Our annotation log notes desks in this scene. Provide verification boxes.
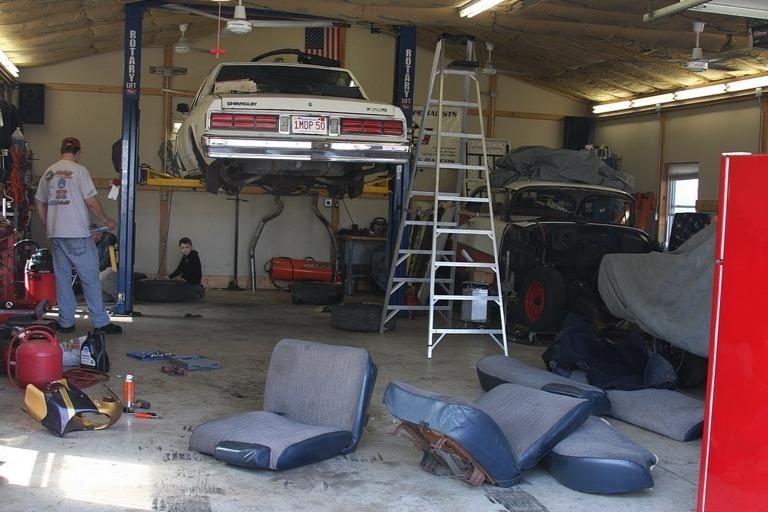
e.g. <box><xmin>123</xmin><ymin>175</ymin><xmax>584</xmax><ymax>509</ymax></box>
<box><xmin>336</xmin><ymin>235</ymin><xmax>390</xmax><ymax>297</ymax></box>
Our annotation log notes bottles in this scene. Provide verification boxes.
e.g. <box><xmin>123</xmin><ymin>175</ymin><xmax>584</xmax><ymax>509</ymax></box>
<box><xmin>89</xmin><ymin>227</ymin><xmax>112</xmax><ymax>236</ymax></box>
<box><xmin>405</xmin><ymin>283</ymin><xmax>418</xmax><ymax>319</ymax></box>
<box><xmin>123</xmin><ymin>373</ymin><xmax>135</xmax><ymax>413</ymax></box>
<box><xmin>336</xmin><ymin>270</ymin><xmax>342</xmax><ymax>285</ymax></box>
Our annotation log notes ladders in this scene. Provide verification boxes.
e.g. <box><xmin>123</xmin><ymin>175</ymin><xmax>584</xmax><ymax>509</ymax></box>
<box><xmin>378</xmin><ymin>33</ymin><xmax>508</xmax><ymax>358</ymax></box>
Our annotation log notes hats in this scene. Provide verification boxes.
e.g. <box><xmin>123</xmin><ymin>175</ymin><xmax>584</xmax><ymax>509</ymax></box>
<box><xmin>62</xmin><ymin>137</ymin><xmax>82</xmax><ymax>147</ymax></box>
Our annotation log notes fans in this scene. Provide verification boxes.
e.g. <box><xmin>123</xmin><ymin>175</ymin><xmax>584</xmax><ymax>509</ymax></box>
<box><xmin>142</xmin><ymin>24</ymin><xmax>209</xmax><ymax>53</ymax></box>
<box><xmin>641</xmin><ymin>21</ymin><xmax>757</xmax><ymax>72</ymax></box>
<box><xmin>163</xmin><ymin>0</ymin><xmax>333</xmax><ymax>34</ymax></box>
<box><xmin>461</xmin><ymin>43</ymin><xmax>530</xmax><ymax>74</ymax></box>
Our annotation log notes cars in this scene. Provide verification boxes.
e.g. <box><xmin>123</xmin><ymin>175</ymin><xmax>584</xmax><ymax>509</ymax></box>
<box><xmin>450</xmin><ymin>182</ymin><xmax>656</xmax><ymax>333</ymax></box>
<box><xmin>169</xmin><ymin>61</ymin><xmax>413</xmax><ymax>200</ymax></box>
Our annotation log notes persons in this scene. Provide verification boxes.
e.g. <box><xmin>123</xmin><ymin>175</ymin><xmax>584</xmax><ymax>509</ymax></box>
<box><xmin>88</xmin><ymin>223</ymin><xmax>120</xmax><ymax>300</ymax></box>
<box><xmin>166</xmin><ymin>237</ymin><xmax>205</xmax><ymax>298</ymax></box>
<box><xmin>34</xmin><ymin>137</ymin><xmax>122</xmax><ymax>335</ymax></box>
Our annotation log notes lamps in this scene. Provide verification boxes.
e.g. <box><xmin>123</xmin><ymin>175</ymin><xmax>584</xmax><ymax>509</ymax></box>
<box><xmin>0</xmin><ymin>54</ymin><xmax>19</xmax><ymax>81</ymax></box>
<box><xmin>590</xmin><ymin>75</ymin><xmax>768</xmax><ymax>117</ymax></box>
<box><xmin>642</xmin><ymin>0</ymin><xmax>714</xmax><ymax>21</ymax></box>
<box><xmin>460</xmin><ymin>0</ymin><xmax>504</xmax><ymax>20</ymax></box>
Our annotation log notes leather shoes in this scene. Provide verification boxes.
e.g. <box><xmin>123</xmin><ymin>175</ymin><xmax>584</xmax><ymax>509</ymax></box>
<box><xmin>61</xmin><ymin>324</ymin><xmax>76</xmax><ymax>333</ymax></box>
<box><xmin>95</xmin><ymin>322</ymin><xmax>121</xmax><ymax>334</ymax></box>
